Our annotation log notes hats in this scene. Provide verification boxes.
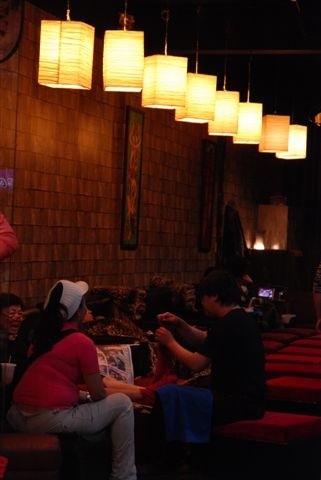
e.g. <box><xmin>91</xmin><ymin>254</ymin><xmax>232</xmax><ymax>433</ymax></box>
<box><xmin>45</xmin><ymin>279</ymin><xmax>89</xmax><ymax>320</ymax></box>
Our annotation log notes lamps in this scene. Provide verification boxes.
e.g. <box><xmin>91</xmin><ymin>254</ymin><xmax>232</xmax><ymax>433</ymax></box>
<box><xmin>207</xmin><ymin>27</ymin><xmax>240</xmax><ymax>136</ymax></box>
<box><xmin>175</xmin><ymin>2</ymin><xmax>217</xmax><ymax>124</ymax></box>
<box><xmin>38</xmin><ymin>0</ymin><xmax>95</xmax><ymax>90</ymax></box>
<box><xmin>102</xmin><ymin>0</ymin><xmax>145</xmax><ymax>92</ymax></box>
<box><xmin>274</xmin><ymin>114</ymin><xmax>308</xmax><ymax>160</ymax></box>
<box><xmin>141</xmin><ymin>10</ymin><xmax>188</xmax><ymax>110</ymax></box>
<box><xmin>232</xmin><ymin>54</ymin><xmax>263</xmax><ymax>145</ymax></box>
<box><xmin>259</xmin><ymin>101</ymin><xmax>292</xmax><ymax>153</ymax></box>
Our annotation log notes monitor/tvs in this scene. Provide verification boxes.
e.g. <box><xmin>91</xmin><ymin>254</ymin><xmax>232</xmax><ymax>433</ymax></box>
<box><xmin>258</xmin><ymin>287</ymin><xmax>275</xmax><ymax>301</ymax></box>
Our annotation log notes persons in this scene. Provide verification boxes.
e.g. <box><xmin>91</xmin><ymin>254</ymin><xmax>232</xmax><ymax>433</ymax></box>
<box><xmin>154</xmin><ymin>270</ymin><xmax>268</xmax><ymax>473</ymax></box>
<box><xmin>0</xmin><ymin>292</ymin><xmax>24</xmax><ymax>425</ymax></box>
<box><xmin>81</xmin><ymin>310</ymin><xmax>190</xmax><ymax>405</ymax></box>
<box><xmin>8</xmin><ymin>280</ymin><xmax>137</xmax><ymax>480</ymax></box>
<box><xmin>312</xmin><ymin>262</ymin><xmax>321</xmax><ymax>330</ymax></box>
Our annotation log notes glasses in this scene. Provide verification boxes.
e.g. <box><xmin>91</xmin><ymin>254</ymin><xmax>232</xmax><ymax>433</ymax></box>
<box><xmin>0</xmin><ymin>312</ymin><xmax>25</xmax><ymax>321</ymax></box>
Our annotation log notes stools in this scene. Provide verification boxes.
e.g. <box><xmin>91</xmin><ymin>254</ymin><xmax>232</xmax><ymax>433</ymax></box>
<box><xmin>0</xmin><ymin>327</ymin><xmax>321</xmax><ymax>480</ymax></box>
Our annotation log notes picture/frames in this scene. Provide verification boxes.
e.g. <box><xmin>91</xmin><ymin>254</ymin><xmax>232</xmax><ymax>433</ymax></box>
<box><xmin>198</xmin><ymin>138</ymin><xmax>217</xmax><ymax>252</ymax></box>
<box><xmin>120</xmin><ymin>105</ymin><xmax>145</xmax><ymax>249</ymax></box>
